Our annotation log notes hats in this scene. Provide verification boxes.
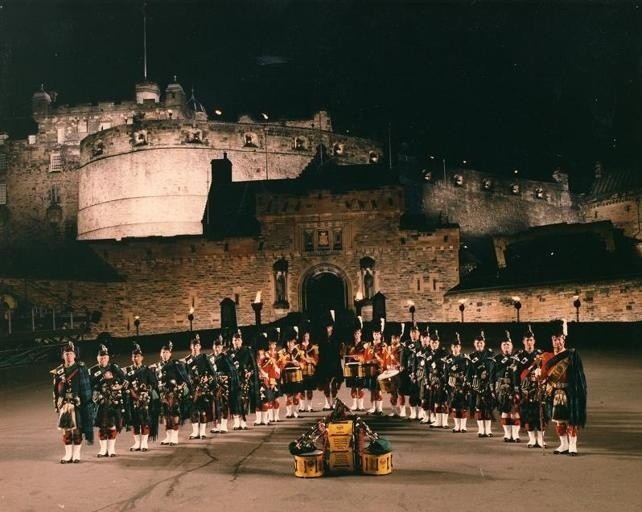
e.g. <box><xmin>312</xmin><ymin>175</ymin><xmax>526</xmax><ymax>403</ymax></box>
<box><xmin>551</xmin><ymin>326</ymin><xmax>563</xmax><ymax>336</ymax></box>
<box><xmin>132</xmin><ymin>348</ymin><xmax>142</xmax><ymax>355</ymax></box>
<box><xmin>355</xmin><ymin>326</ymin><xmax>401</xmax><ymax>336</ymax></box>
<box><xmin>523</xmin><ymin>331</ymin><xmax>534</xmax><ymax>338</ymax></box>
<box><xmin>476</xmin><ymin>335</ymin><xmax>483</xmax><ymax>341</ymax></box>
<box><xmin>502</xmin><ymin>338</ymin><xmax>511</xmax><ymax>342</ymax></box>
<box><xmin>162</xmin><ymin>345</ymin><xmax>170</xmax><ymax>350</ymax></box>
<box><xmin>257</xmin><ymin>320</ymin><xmax>332</xmax><ymax>349</ymax></box>
<box><xmin>410</xmin><ymin>326</ymin><xmax>439</xmax><ymax>341</ymax></box>
<box><xmin>213</xmin><ymin>340</ymin><xmax>222</xmax><ymax>345</ymax></box>
<box><xmin>190</xmin><ymin>339</ymin><xmax>200</xmax><ymax>344</ymax></box>
<box><xmin>233</xmin><ymin>333</ymin><xmax>241</xmax><ymax>338</ymax></box>
<box><xmin>97</xmin><ymin>349</ymin><xmax>108</xmax><ymax>356</ymax></box>
<box><xmin>454</xmin><ymin>339</ymin><xmax>461</xmax><ymax>345</ymax></box>
<box><xmin>62</xmin><ymin>343</ymin><xmax>74</xmax><ymax>352</ymax></box>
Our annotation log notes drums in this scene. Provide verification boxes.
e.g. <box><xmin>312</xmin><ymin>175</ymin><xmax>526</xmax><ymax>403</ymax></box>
<box><xmin>344</xmin><ymin>362</ymin><xmax>359</xmax><ymax>379</ymax></box>
<box><xmin>293</xmin><ymin>449</ymin><xmax>324</xmax><ymax>479</ymax></box>
<box><xmin>358</xmin><ymin>361</ymin><xmax>379</xmax><ymax>379</ymax></box>
<box><xmin>301</xmin><ymin>359</ymin><xmax>317</xmax><ymax>377</ymax></box>
<box><xmin>284</xmin><ymin>366</ymin><xmax>304</xmax><ymax>386</ymax></box>
<box><xmin>377</xmin><ymin>368</ymin><xmax>401</xmax><ymax>395</ymax></box>
<box><xmin>358</xmin><ymin>447</ymin><xmax>394</xmax><ymax>476</ymax></box>
<box><xmin>323</xmin><ymin>419</ymin><xmax>365</xmax><ymax>477</ymax></box>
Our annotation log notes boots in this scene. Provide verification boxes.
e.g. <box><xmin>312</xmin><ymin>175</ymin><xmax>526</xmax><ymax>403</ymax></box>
<box><xmin>527</xmin><ymin>430</ymin><xmax>536</xmax><ymax>448</ymax></box>
<box><xmin>527</xmin><ymin>430</ymin><xmax>547</xmax><ymax>448</ymax></box>
<box><xmin>350</xmin><ymin>396</ymin><xmax>492</xmax><ymax>443</ymax></box>
<box><xmin>254</xmin><ymin>408</ymin><xmax>281</xmax><ymax>425</ymax></box>
<box><xmin>568</xmin><ymin>433</ymin><xmax>577</xmax><ymax>456</ymax></box>
<box><xmin>72</xmin><ymin>444</ymin><xmax>82</xmax><ymax>463</ymax></box>
<box><xmin>107</xmin><ymin>438</ymin><xmax>117</xmax><ymax>457</ymax></box>
<box><xmin>129</xmin><ymin>433</ymin><xmax>140</xmax><ymax>451</ymax></box>
<box><xmin>285</xmin><ymin>399</ymin><xmax>313</xmax><ymax>418</ymax></box>
<box><xmin>189</xmin><ymin>422</ymin><xmax>208</xmax><ymax>439</ymax></box>
<box><xmin>233</xmin><ymin>413</ymin><xmax>248</xmax><ymax>430</ymax></box>
<box><xmin>512</xmin><ymin>425</ymin><xmax>520</xmax><ymax>443</ymax></box>
<box><xmin>537</xmin><ymin>430</ymin><xmax>547</xmax><ymax>448</ymax></box>
<box><xmin>323</xmin><ymin>395</ymin><xmax>336</xmax><ymax>410</ymax></box>
<box><xmin>554</xmin><ymin>435</ymin><xmax>568</xmax><ymax>454</ymax></box>
<box><xmin>97</xmin><ymin>439</ymin><xmax>107</xmax><ymax>457</ymax></box>
<box><xmin>141</xmin><ymin>433</ymin><xmax>149</xmax><ymax>452</ymax></box>
<box><xmin>502</xmin><ymin>424</ymin><xmax>511</xmax><ymax>441</ymax></box>
<box><xmin>210</xmin><ymin>417</ymin><xmax>228</xmax><ymax>433</ymax></box>
<box><xmin>160</xmin><ymin>429</ymin><xmax>178</xmax><ymax>445</ymax></box>
<box><xmin>61</xmin><ymin>444</ymin><xmax>72</xmax><ymax>464</ymax></box>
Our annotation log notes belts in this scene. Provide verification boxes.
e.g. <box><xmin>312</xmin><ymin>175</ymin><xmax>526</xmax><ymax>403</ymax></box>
<box><xmin>553</xmin><ymin>383</ymin><xmax>569</xmax><ymax>388</ymax></box>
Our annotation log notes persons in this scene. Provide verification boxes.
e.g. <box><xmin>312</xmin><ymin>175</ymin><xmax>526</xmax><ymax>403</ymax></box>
<box><xmin>148</xmin><ymin>333</ymin><xmax>258</xmax><ymax>446</ymax></box>
<box><xmin>468</xmin><ymin>331</ymin><xmax>586</xmax><ymax>456</ymax></box>
<box><xmin>49</xmin><ymin>345</ymin><xmax>92</xmax><ymax>463</ymax></box>
<box><xmin>87</xmin><ymin>343</ymin><xmax>160</xmax><ymax>457</ymax></box>
<box><xmin>316</xmin><ymin>324</ymin><xmax>345</xmax><ymax>411</ymax></box>
<box><xmin>254</xmin><ymin>331</ymin><xmax>319</xmax><ymax>425</ymax></box>
<box><xmin>345</xmin><ymin>329</ymin><xmax>406</xmax><ymax>418</ymax></box>
<box><xmin>397</xmin><ymin>325</ymin><xmax>471</xmax><ymax>432</ymax></box>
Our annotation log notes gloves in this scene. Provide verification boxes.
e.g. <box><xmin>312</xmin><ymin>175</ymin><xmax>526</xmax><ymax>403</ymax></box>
<box><xmin>534</xmin><ymin>367</ymin><xmax>541</xmax><ymax>378</ymax></box>
